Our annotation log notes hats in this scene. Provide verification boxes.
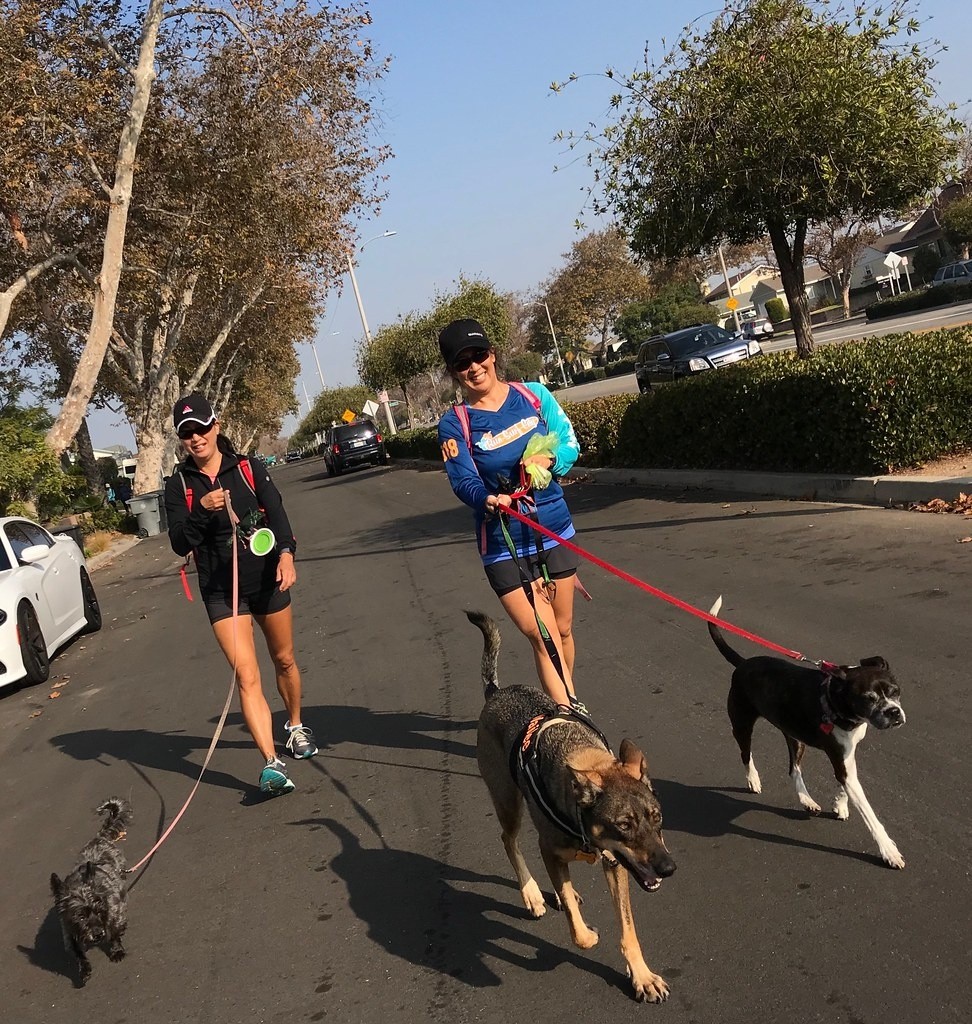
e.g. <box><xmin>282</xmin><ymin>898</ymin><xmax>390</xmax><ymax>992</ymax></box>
<box><xmin>438</xmin><ymin>318</ymin><xmax>490</xmax><ymax>368</ymax></box>
<box><xmin>173</xmin><ymin>395</ymin><xmax>216</xmax><ymax>434</ymax></box>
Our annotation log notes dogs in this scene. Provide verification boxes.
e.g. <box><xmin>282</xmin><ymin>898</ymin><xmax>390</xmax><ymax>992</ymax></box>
<box><xmin>461</xmin><ymin>608</ymin><xmax>677</xmax><ymax>1005</ymax></box>
<box><xmin>49</xmin><ymin>795</ymin><xmax>133</xmax><ymax>984</ymax></box>
<box><xmin>707</xmin><ymin>593</ymin><xmax>908</xmax><ymax>872</ymax></box>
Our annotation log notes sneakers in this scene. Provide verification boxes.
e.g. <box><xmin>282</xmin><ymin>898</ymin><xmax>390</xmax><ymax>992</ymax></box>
<box><xmin>284</xmin><ymin>720</ymin><xmax>319</xmax><ymax>760</ymax></box>
<box><xmin>258</xmin><ymin>758</ymin><xmax>296</xmax><ymax>797</ymax></box>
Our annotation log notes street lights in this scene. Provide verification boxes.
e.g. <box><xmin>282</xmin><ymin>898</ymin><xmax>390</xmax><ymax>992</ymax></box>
<box><xmin>523</xmin><ymin>301</ymin><xmax>568</xmax><ymax>388</ymax></box>
<box><xmin>300</xmin><ymin>228</ymin><xmax>398</xmax><ymax>434</ymax></box>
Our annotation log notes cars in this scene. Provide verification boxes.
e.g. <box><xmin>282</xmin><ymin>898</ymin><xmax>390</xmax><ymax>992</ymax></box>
<box><xmin>0</xmin><ymin>516</ymin><xmax>102</xmax><ymax>690</ymax></box>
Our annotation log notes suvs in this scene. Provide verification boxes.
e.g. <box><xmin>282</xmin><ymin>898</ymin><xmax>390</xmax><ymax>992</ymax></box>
<box><xmin>634</xmin><ymin>321</ymin><xmax>763</xmax><ymax>395</ymax></box>
<box><xmin>323</xmin><ymin>419</ymin><xmax>388</xmax><ymax>474</ymax></box>
<box><xmin>284</xmin><ymin>448</ymin><xmax>301</xmax><ymax>462</ymax></box>
<box><xmin>742</xmin><ymin>319</ymin><xmax>774</xmax><ymax>341</ymax></box>
<box><xmin>933</xmin><ymin>258</ymin><xmax>972</xmax><ymax>285</ymax></box>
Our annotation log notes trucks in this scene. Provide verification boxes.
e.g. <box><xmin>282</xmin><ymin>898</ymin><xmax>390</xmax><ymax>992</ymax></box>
<box><xmin>256</xmin><ymin>454</ymin><xmax>276</xmax><ymax>467</ymax></box>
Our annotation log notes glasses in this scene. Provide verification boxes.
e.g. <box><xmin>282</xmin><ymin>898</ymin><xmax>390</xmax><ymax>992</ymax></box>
<box><xmin>176</xmin><ymin>418</ymin><xmax>215</xmax><ymax>439</ymax></box>
<box><xmin>450</xmin><ymin>346</ymin><xmax>490</xmax><ymax>372</ymax></box>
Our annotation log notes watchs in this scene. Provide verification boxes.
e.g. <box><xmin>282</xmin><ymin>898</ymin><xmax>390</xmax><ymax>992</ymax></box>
<box><xmin>280</xmin><ymin>547</ymin><xmax>295</xmax><ymax>557</ymax></box>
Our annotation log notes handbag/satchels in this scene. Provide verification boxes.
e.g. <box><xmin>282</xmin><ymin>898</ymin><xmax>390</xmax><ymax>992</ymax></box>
<box><xmin>520</xmin><ymin>430</ymin><xmax>559</xmax><ymax>489</ymax></box>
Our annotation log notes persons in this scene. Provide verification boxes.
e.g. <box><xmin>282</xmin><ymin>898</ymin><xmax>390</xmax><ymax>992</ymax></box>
<box><xmin>117</xmin><ymin>480</ymin><xmax>131</xmax><ymax>516</ymax></box>
<box><xmin>164</xmin><ymin>396</ymin><xmax>318</xmax><ymax>795</ymax></box>
<box><xmin>439</xmin><ymin>319</ymin><xmax>580</xmax><ymax>714</ymax></box>
<box><xmin>104</xmin><ymin>483</ymin><xmax>117</xmax><ymax>514</ymax></box>
